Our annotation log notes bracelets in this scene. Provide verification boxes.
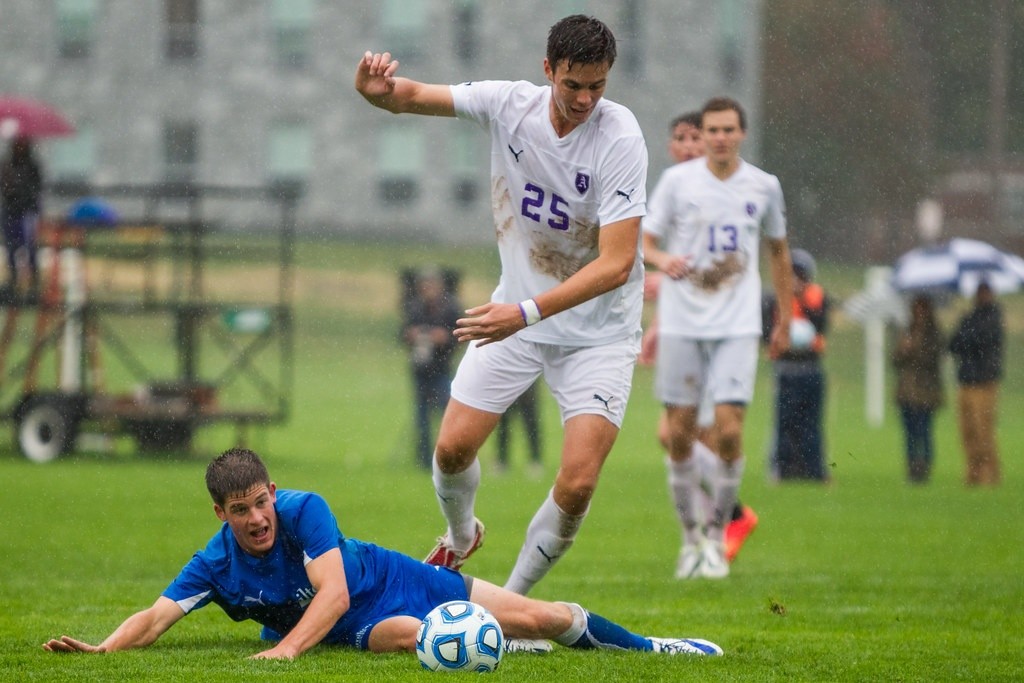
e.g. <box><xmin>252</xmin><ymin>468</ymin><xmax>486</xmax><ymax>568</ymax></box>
<box><xmin>518</xmin><ymin>298</ymin><xmax>542</xmax><ymax>327</ymax></box>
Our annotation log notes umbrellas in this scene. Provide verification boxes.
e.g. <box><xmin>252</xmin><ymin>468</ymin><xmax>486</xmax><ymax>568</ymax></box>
<box><xmin>0</xmin><ymin>94</ymin><xmax>79</xmax><ymax>138</ymax></box>
<box><xmin>886</xmin><ymin>236</ymin><xmax>1024</xmax><ymax>311</ymax></box>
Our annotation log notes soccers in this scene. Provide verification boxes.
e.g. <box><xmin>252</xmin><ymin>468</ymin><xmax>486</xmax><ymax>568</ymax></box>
<box><xmin>415</xmin><ymin>599</ymin><xmax>506</xmax><ymax>674</ymax></box>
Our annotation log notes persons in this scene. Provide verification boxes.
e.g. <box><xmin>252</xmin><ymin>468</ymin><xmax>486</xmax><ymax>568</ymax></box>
<box><xmin>398</xmin><ymin>269</ymin><xmax>472</xmax><ymax>470</ymax></box>
<box><xmin>641</xmin><ymin>96</ymin><xmax>796</xmax><ymax>579</ymax></box>
<box><xmin>42</xmin><ymin>447</ymin><xmax>724</xmax><ymax>660</ymax></box>
<box><xmin>641</xmin><ymin>111</ymin><xmax>759</xmax><ymax>566</ymax></box>
<box><xmin>892</xmin><ymin>296</ymin><xmax>945</xmax><ymax>480</ymax></box>
<box><xmin>0</xmin><ymin>136</ymin><xmax>42</xmax><ymax>297</ymax></box>
<box><xmin>761</xmin><ymin>247</ymin><xmax>831</xmax><ymax>482</ymax></box>
<box><xmin>949</xmin><ymin>283</ymin><xmax>1006</xmax><ymax>487</ymax></box>
<box><xmin>498</xmin><ymin>382</ymin><xmax>547</xmax><ymax>472</ymax></box>
<box><xmin>355</xmin><ymin>13</ymin><xmax>649</xmax><ymax>596</ymax></box>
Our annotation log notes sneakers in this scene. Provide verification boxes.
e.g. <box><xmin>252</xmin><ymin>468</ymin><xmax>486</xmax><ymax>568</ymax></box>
<box><xmin>646</xmin><ymin>635</ymin><xmax>724</xmax><ymax>658</ymax></box>
<box><xmin>676</xmin><ymin>539</ymin><xmax>728</xmax><ymax>580</ymax></box>
<box><xmin>506</xmin><ymin>639</ymin><xmax>553</xmax><ymax>653</ymax></box>
<box><xmin>423</xmin><ymin>516</ymin><xmax>484</xmax><ymax>573</ymax></box>
<box><xmin>723</xmin><ymin>505</ymin><xmax>757</xmax><ymax>556</ymax></box>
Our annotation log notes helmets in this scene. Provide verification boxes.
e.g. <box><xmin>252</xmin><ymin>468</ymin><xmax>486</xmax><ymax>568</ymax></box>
<box><xmin>790</xmin><ymin>248</ymin><xmax>816</xmax><ymax>282</ymax></box>
